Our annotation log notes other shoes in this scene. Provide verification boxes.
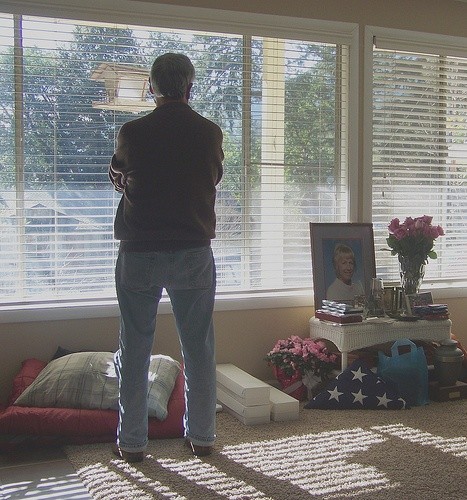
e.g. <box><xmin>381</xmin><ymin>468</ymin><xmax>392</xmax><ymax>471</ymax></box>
<box><xmin>187</xmin><ymin>439</ymin><xmax>211</xmax><ymax>456</ymax></box>
<box><xmin>112</xmin><ymin>447</ymin><xmax>143</xmax><ymax>462</ymax></box>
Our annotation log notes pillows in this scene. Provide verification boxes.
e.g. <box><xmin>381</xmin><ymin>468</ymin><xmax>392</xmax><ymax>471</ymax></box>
<box><xmin>0</xmin><ymin>346</ymin><xmax>185</xmax><ymax>438</ymax></box>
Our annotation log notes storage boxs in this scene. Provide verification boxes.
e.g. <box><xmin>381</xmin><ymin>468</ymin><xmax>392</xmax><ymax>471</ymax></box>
<box><xmin>216</xmin><ymin>364</ymin><xmax>300</xmax><ymax>427</ymax></box>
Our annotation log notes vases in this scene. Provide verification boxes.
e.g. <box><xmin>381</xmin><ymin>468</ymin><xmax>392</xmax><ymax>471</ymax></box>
<box><xmin>278</xmin><ymin>375</ymin><xmax>305</xmax><ymax>400</ymax></box>
<box><xmin>399</xmin><ymin>260</ymin><xmax>425</xmax><ymax>306</ymax></box>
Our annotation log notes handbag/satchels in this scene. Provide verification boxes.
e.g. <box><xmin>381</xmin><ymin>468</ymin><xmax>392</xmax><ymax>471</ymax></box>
<box><xmin>378</xmin><ymin>338</ymin><xmax>429</xmax><ymax>406</ymax></box>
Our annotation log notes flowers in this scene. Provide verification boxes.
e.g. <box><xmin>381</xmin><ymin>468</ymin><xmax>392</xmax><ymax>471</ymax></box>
<box><xmin>380</xmin><ymin>216</ymin><xmax>445</xmax><ymax>296</ymax></box>
<box><xmin>263</xmin><ymin>335</ymin><xmax>336</xmax><ymax>382</ymax></box>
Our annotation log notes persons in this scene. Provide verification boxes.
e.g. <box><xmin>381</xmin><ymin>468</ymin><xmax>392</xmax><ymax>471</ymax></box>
<box><xmin>109</xmin><ymin>52</ymin><xmax>224</xmax><ymax>461</ymax></box>
<box><xmin>326</xmin><ymin>242</ymin><xmax>366</xmax><ymax>300</ymax></box>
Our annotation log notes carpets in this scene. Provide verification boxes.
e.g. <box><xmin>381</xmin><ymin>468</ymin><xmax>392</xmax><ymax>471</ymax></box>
<box><xmin>64</xmin><ymin>398</ymin><xmax>467</xmax><ymax>500</ymax></box>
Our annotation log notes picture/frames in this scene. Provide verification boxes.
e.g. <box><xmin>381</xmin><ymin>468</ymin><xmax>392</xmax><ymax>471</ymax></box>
<box><xmin>309</xmin><ymin>222</ymin><xmax>376</xmax><ymax>312</ymax></box>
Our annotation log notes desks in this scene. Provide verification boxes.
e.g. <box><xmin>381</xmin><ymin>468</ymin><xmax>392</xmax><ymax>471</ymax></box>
<box><xmin>308</xmin><ymin>316</ymin><xmax>452</xmax><ymax>400</ymax></box>
